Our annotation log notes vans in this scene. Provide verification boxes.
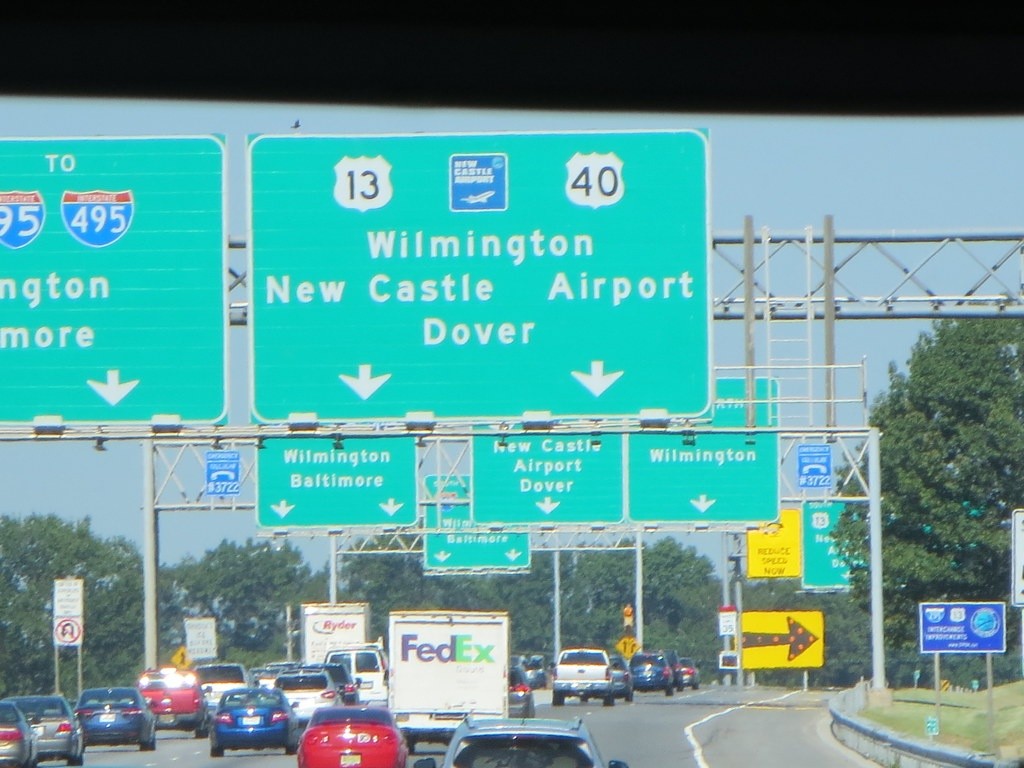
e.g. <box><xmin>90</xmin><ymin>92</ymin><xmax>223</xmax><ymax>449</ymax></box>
<box><xmin>325</xmin><ymin>643</ymin><xmax>390</xmax><ymax>704</ymax></box>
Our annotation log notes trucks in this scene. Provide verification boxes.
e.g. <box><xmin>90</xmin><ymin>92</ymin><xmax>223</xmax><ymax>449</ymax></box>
<box><xmin>386</xmin><ymin>610</ymin><xmax>512</xmax><ymax>752</ymax></box>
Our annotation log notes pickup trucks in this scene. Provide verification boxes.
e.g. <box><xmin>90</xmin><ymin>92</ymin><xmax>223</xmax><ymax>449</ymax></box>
<box><xmin>134</xmin><ymin>666</ymin><xmax>212</xmax><ymax>739</ymax></box>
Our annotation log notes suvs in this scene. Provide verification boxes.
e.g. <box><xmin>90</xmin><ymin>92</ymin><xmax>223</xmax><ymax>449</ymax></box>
<box><xmin>413</xmin><ymin>715</ymin><xmax>629</xmax><ymax>768</ymax></box>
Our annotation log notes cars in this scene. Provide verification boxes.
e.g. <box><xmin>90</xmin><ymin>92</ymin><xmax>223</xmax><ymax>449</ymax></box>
<box><xmin>3</xmin><ymin>696</ymin><xmax>85</xmax><ymax>767</ymax></box>
<box><xmin>246</xmin><ymin>643</ymin><xmax>364</xmax><ymax>725</ymax></box>
<box><xmin>206</xmin><ymin>687</ymin><xmax>300</xmax><ymax>757</ymax></box>
<box><xmin>506</xmin><ymin>640</ymin><xmax>701</xmax><ymax>721</ymax></box>
<box><xmin>296</xmin><ymin>705</ymin><xmax>409</xmax><ymax>768</ymax></box>
<box><xmin>69</xmin><ymin>687</ymin><xmax>158</xmax><ymax>753</ymax></box>
<box><xmin>0</xmin><ymin>701</ymin><xmax>43</xmax><ymax>768</ymax></box>
<box><xmin>191</xmin><ymin>664</ymin><xmax>255</xmax><ymax>711</ymax></box>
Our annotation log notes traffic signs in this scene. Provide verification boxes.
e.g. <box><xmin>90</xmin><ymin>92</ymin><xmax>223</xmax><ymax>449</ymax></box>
<box><xmin>628</xmin><ymin>375</ymin><xmax>782</xmax><ymax>524</ymax></box>
<box><xmin>256</xmin><ymin>434</ymin><xmax>420</xmax><ymax>530</ymax></box>
<box><xmin>0</xmin><ymin>136</ymin><xmax>229</xmax><ymax>427</ymax></box>
<box><xmin>242</xmin><ymin>133</ymin><xmax>713</xmax><ymax>422</ymax></box>
<box><xmin>422</xmin><ymin>475</ymin><xmax>532</xmax><ymax>570</ymax></box>
<box><xmin>469</xmin><ymin>420</ymin><xmax>625</xmax><ymax>527</ymax></box>
